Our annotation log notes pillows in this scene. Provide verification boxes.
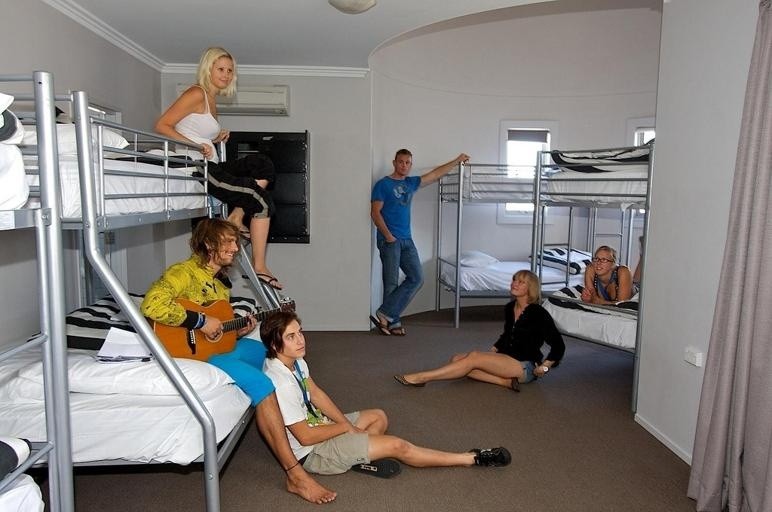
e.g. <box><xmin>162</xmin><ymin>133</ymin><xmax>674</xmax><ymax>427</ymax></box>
<box><xmin>446</xmin><ymin>245</ymin><xmax>504</xmax><ymax>266</ymax></box>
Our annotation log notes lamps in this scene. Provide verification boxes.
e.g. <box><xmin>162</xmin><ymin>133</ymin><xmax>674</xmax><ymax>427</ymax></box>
<box><xmin>326</xmin><ymin>1</ymin><xmax>377</xmax><ymax>16</ymax></box>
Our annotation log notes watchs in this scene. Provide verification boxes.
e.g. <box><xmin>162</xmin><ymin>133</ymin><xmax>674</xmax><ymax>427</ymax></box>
<box><xmin>541</xmin><ymin>365</ymin><xmax>549</xmax><ymax>373</ymax></box>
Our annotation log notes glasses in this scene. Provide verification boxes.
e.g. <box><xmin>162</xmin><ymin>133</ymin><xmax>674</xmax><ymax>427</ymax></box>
<box><xmin>592</xmin><ymin>258</ymin><xmax>614</xmax><ymax>263</ymax></box>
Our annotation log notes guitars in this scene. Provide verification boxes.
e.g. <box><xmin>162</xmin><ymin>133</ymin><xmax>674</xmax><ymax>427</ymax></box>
<box><xmin>147</xmin><ymin>299</ymin><xmax>295</xmax><ymax>361</ymax></box>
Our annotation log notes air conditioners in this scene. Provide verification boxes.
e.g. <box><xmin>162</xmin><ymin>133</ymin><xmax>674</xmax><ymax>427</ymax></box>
<box><xmin>174</xmin><ymin>79</ymin><xmax>294</xmax><ymax>120</ymax></box>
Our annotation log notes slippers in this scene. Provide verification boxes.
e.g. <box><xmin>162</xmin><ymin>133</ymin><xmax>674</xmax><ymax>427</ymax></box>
<box><xmin>369</xmin><ymin>315</ymin><xmax>390</xmax><ymax>336</ymax></box>
<box><xmin>242</xmin><ymin>271</ymin><xmax>282</xmax><ymax>290</ymax></box>
<box><xmin>389</xmin><ymin>327</ymin><xmax>406</xmax><ymax>336</ymax></box>
<box><xmin>238</xmin><ymin>227</ymin><xmax>252</xmax><ymax>242</ymax></box>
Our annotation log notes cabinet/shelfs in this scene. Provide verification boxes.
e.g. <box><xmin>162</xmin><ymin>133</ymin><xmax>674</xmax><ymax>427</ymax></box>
<box><xmin>189</xmin><ymin>130</ymin><xmax>313</xmax><ymax>244</ymax></box>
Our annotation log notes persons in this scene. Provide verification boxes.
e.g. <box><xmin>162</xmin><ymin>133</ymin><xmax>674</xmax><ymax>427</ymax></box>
<box><xmin>155</xmin><ymin>48</ymin><xmax>282</xmax><ymax>292</ymax></box>
<box><xmin>394</xmin><ymin>270</ymin><xmax>566</xmax><ymax>392</ymax></box>
<box><xmin>368</xmin><ymin>148</ymin><xmax>472</xmax><ymax>336</ymax></box>
<box><xmin>140</xmin><ymin>215</ymin><xmax>338</xmax><ymax>505</ymax></box>
<box><xmin>259</xmin><ymin>312</ymin><xmax>511</xmax><ymax>479</ymax></box>
<box><xmin>581</xmin><ymin>237</ymin><xmax>644</xmax><ymax>304</ymax></box>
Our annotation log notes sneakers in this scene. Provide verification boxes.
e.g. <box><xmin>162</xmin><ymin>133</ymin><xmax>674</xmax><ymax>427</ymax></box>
<box><xmin>468</xmin><ymin>446</ymin><xmax>512</xmax><ymax>468</ymax></box>
<box><xmin>353</xmin><ymin>459</ymin><xmax>401</xmax><ymax>479</ymax></box>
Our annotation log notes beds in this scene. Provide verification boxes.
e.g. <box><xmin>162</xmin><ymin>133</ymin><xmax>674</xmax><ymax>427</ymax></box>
<box><xmin>1</xmin><ymin>69</ymin><xmax>295</xmax><ymax>511</ymax></box>
<box><xmin>436</xmin><ymin>156</ymin><xmax>635</xmax><ymax>330</ymax></box>
<box><xmin>3</xmin><ymin>73</ymin><xmax>80</xmax><ymax>509</ymax></box>
<box><xmin>530</xmin><ymin>142</ymin><xmax>653</xmax><ymax>413</ymax></box>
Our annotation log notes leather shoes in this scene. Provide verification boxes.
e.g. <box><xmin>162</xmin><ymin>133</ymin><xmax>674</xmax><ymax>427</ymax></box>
<box><xmin>508</xmin><ymin>377</ymin><xmax>521</xmax><ymax>391</ymax></box>
<box><xmin>394</xmin><ymin>374</ymin><xmax>426</xmax><ymax>387</ymax></box>
